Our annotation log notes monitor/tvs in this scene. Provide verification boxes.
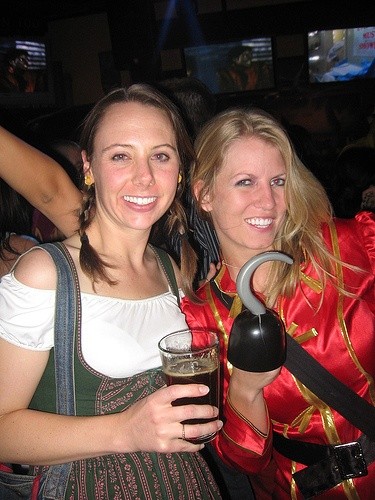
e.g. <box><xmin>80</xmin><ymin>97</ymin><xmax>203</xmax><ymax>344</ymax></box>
<box><xmin>304</xmin><ymin>26</ymin><xmax>375</xmax><ymax>87</ymax></box>
<box><xmin>180</xmin><ymin>35</ymin><xmax>276</xmax><ymax>103</ymax></box>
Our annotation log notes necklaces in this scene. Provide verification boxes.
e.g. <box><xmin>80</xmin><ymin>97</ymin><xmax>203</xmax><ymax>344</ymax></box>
<box><xmin>224</xmin><ymin>262</ymin><xmax>241</xmax><ymax>270</ymax></box>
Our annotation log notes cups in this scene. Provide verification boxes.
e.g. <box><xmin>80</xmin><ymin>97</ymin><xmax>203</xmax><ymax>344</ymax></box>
<box><xmin>158</xmin><ymin>329</ymin><xmax>220</xmax><ymax>444</ymax></box>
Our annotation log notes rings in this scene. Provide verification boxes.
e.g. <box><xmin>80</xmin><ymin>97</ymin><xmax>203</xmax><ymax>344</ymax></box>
<box><xmin>181</xmin><ymin>424</ymin><xmax>186</xmax><ymax>440</ymax></box>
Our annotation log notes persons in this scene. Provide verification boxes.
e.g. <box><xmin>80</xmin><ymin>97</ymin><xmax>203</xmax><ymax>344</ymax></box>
<box><xmin>0</xmin><ymin>49</ymin><xmax>36</xmax><ymax>93</ymax></box>
<box><xmin>0</xmin><ymin>78</ymin><xmax>375</xmax><ymax>290</ymax></box>
<box><xmin>0</xmin><ymin>85</ymin><xmax>223</xmax><ymax>500</ymax></box>
<box><xmin>216</xmin><ymin>46</ymin><xmax>263</xmax><ymax>93</ymax></box>
<box><xmin>179</xmin><ymin>107</ymin><xmax>375</xmax><ymax>500</ymax></box>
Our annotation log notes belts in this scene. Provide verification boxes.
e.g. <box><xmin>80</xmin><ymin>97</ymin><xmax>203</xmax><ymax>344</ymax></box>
<box><xmin>272</xmin><ymin>430</ymin><xmax>374</xmax><ymax>500</ymax></box>
